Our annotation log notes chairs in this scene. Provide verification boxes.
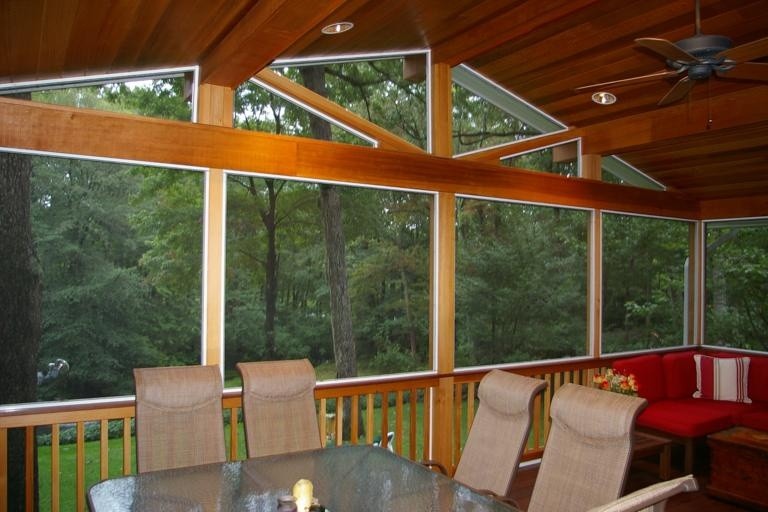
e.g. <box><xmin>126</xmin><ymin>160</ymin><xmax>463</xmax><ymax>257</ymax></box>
<box><xmin>131</xmin><ymin>357</ymin><xmax>324</xmax><ymax>474</ymax></box>
<box><xmin>419</xmin><ymin>369</ymin><xmax>699</xmax><ymax>512</ymax></box>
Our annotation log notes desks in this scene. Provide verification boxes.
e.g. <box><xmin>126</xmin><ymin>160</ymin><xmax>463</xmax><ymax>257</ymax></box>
<box><xmin>85</xmin><ymin>441</ymin><xmax>522</xmax><ymax>512</ymax></box>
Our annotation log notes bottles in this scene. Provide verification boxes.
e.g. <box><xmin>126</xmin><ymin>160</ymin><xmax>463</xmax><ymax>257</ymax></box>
<box><xmin>276</xmin><ymin>495</ymin><xmax>298</xmax><ymax>511</ymax></box>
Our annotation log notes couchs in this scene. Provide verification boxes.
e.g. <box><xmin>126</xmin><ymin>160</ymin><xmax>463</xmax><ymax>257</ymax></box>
<box><xmin>611</xmin><ymin>350</ymin><xmax>768</xmax><ymax>476</ymax></box>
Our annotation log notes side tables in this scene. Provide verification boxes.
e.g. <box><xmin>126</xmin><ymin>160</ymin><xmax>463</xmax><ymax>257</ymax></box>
<box><xmin>705</xmin><ymin>426</ymin><xmax>768</xmax><ymax>510</ymax></box>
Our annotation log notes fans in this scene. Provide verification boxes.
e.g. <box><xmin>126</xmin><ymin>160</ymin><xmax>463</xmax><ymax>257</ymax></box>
<box><xmin>572</xmin><ymin>1</ymin><xmax>768</xmax><ymax>107</ymax></box>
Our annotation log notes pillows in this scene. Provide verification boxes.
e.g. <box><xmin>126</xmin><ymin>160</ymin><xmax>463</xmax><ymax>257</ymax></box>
<box><xmin>692</xmin><ymin>355</ymin><xmax>755</xmax><ymax>404</ymax></box>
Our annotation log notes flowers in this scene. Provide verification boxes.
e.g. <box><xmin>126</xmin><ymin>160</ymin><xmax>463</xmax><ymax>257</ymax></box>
<box><xmin>593</xmin><ymin>368</ymin><xmax>639</xmax><ymax>397</ymax></box>
